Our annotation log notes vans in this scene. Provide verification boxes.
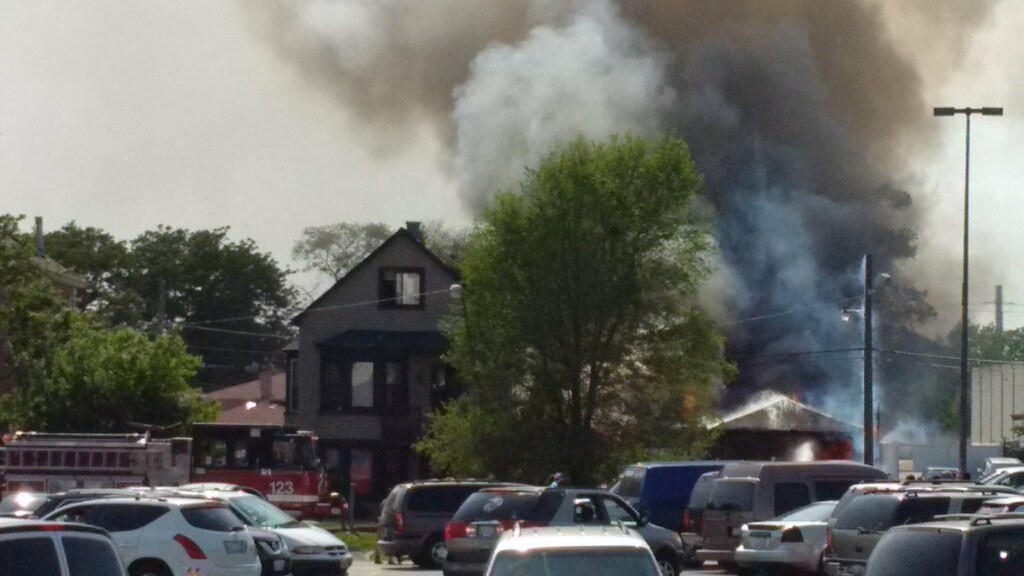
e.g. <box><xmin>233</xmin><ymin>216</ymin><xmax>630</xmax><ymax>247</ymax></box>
<box><xmin>609</xmin><ymin>459</ymin><xmax>892</xmax><ymax>563</ymax></box>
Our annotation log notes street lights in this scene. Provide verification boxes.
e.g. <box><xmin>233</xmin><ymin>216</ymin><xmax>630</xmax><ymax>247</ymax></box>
<box><xmin>933</xmin><ymin>106</ymin><xmax>1003</xmax><ymax>481</ymax></box>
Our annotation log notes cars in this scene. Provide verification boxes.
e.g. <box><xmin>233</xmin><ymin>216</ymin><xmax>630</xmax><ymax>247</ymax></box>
<box><xmin>735</xmin><ymin>501</ymin><xmax>840</xmax><ymax>575</ymax></box>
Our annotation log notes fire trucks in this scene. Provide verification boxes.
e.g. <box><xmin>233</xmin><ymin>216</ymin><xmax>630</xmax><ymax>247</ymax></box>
<box><xmin>0</xmin><ymin>423</ymin><xmax>349</xmax><ymax>521</ymax></box>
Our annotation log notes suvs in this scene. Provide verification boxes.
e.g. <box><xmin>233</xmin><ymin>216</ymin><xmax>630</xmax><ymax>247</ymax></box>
<box><xmin>483</xmin><ymin>521</ymin><xmax>663</xmax><ymax>576</ymax></box>
<box><xmin>376</xmin><ymin>477</ymin><xmax>501</xmax><ymax>570</ymax></box>
<box><xmin>822</xmin><ymin>456</ymin><xmax>1024</xmax><ymax>576</ymax></box>
<box><xmin>0</xmin><ymin>482</ymin><xmax>352</xmax><ymax>576</ymax></box>
<box><xmin>443</xmin><ymin>484</ymin><xmax>686</xmax><ymax>576</ymax></box>
